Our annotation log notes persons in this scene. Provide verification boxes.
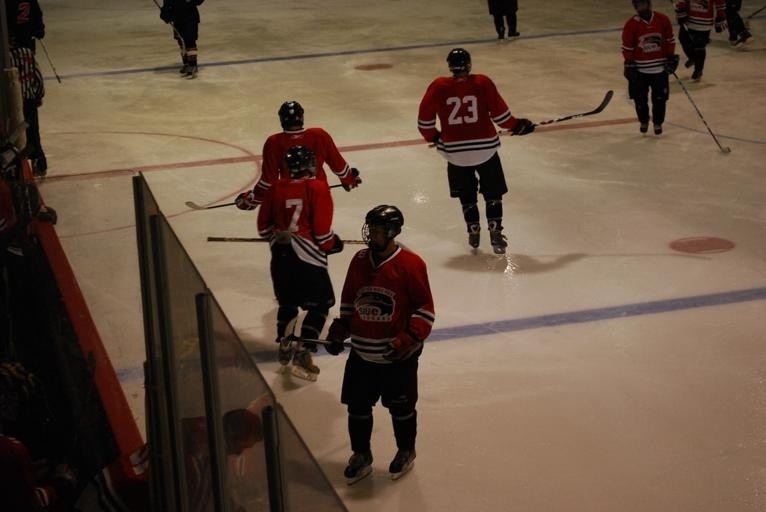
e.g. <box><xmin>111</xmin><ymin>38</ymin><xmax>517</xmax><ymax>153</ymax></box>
<box><xmin>77</xmin><ymin>395</ymin><xmax>278</xmax><ymax>511</ymax></box>
<box><xmin>0</xmin><ymin>1</ymin><xmax>51</xmax><ymax>181</ymax></box>
<box><xmin>484</xmin><ymin>1</ymin><xmax>520</xmax><ymax>41</ymax></box>
<box><xmin>674</xmin><ymin>1</ymin><xmax>728</xmax><ymax>81</ymax></box>
<box><xmin>0</xmin><ymin>118</ymin><xmax>94</xmax><ymax>509</ymax></box>
<box><xmin>252</xmin><ymin>143</ymin><xmax>344</xmax><ymax>385</ymax></box>
<box><xmin>157</xmin><ymin>0</ymin><xmax>206</xmax><ymax>82</ymax></box>
<box><xmin>235</xmin><ymin>97</ymin><xmax>364</xmax><ymax>214</ymax></box>
<box><xmin>320</xmin><ymin>202</ymin><xmax>436</xmax><ymax>486</ymax></box>
<box><xmin>725</xmin><ymin>1</ymin><xmax>755</xmax><ymax>50</ymax></box>
<box><xmin>618</xmin><ymin>1</ymin><xmax>677</xmax><ymax>137</ymax></box>
<box><xmin>416</xmin><ymin>46</ymin><xmax>535</xmax><ymax>258</ymax></box>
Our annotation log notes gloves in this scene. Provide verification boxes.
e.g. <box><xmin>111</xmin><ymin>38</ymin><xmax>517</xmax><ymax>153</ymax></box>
<box><xmin>511</xmin><ymin>118</ymin><xmax>535</xmax><ymax>135</ymax></box>
<box><xmin>675</xmin><ymin>8</ymin><xmax>689</xmax><ymax>25</ymax></box>
<box><xmin>324</xmin><ymin>318</ymin><xmax>351</xmax><ymax>355</ymax></box>
<box><xmin>667</xmin><ymin>53</ymin><xmax>680</xmax><ymax>71</ymax></box>
<box><xmin>35</xmin><ymin>205</ymin><xmax>57</xmax><ymax>225</ymax></box>
<box><xmin>327</xmin><ymin>234</ymin><xmax>343</xmax><ymax>255</ymax></box>
<box><xmin>624</xmin><ymin>67</ymin><xmax>638</xmax><ymax>80</ymax></box>
<box><xmin>235</xmin><ymin>189</ymin><xmax>258</xmax><ymax>210</ymax></box>
<box><xmin>715</xmin><ymin>16</ymin><xmax>728</xmax><ymax>33</ymax></box>
<box><xmin>383</xmin><ymin>328</ymin><xmax>416</xmax><ymax>362</ymax></box>
<box><xmin>341</xmin><ymin>167</ymin><xmax>362</xmax><ymax>191</ymax></box>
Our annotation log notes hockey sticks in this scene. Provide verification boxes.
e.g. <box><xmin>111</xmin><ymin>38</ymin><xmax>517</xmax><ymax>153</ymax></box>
<box><xmin>205</xmin><ymin>236</ymin><xmax>409</xmax><ymax>248</ymax></box>
<box><xmin>184</xmin><ymin>183</ymin><xmax>343</xmax><ymax>210</ymax></box>
<box><xmin>672</xmin><ymin>72</ymin><xmax>731</xmax><ymax>154</ymax></box>
<box><xmin>287</xmin><ymin>318</ymin><xmax>350</xmax><ymax>349</ymax></box>
<box><xmin>429</xmin><ymin>89</ymin><xmax>615</xmax><ymax>149</ymax></box>
<box><xmin>152</xmin><ymin>0</ymin><xmax>185</xmax><ymax>57</ymax></box>
<box><xmin>39</xmin><ymin>38</ymin><xmax>63</xmax><ymax>84</ymax></box>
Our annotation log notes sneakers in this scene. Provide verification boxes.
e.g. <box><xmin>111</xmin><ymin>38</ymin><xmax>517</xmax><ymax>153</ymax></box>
<box><xmin>728</xmin><ymin>32</ymin><xmax>739</xmax><ymax>41</ymax></box>
<box><xmin>692</xmin><ymin>67</ymin><xmax>703</xmax><ymax>78</ymax></box>
<box><xmin>498</xmin><ymin>32</ymin><xmax>504</xmax><ymax>39</ymax></box>
<box><xmin>466</xmin><ymin>222</ymin><xmax>481</xmax><ymax>247</ymax></box>
<box><xmin>508</xmin><ymin>31</ymin><xmax>520</xmax><ymax>37</ymax></box>
<box><xmin>344</xmin><ymin>450</ymin><xmax>373</xmax><ymax>478</ymax></box>
<box><xmin>278</xmin><ymin>343</ymin><xmax>292</xmax><ymax>365</ymax></box>
<box><xmin>640</xmin><ymin>122</ymin><xmax>648</xmax><ymax>132</ymax></box>
<box><xmin>684</xmin><ymin>57</ymin><xmax>694</xmax><ymax>69</ymax></box>
<box><xmin>735</xmin><ymin>30</ymin><xmax>752</xmax><ymax>46</ymax></box>
<box><xmin>292</xmin><ymin>350</ymin><xmax>320</xmax><ymax>374</ymax></box>
<box><xmin>389</xmin><ymin>448</ymin><xmax>416</xmax><ymax>473</ymax></box>
<box><xmin>488</xmin><ymin>220</ymin><xmax>508</xmax><ymax>248</ymax></box>
<box><xmin>654</xmin><ymin>123</ymin><xmax>662</xmax><ymax>134</ymax></box>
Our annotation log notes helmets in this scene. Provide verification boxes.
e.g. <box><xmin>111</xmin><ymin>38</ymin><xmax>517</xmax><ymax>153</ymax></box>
<box><xmin>284</xmin><ymin>145</ymin><xmax>317</xmax><ymax>178</ymax></box>
<box><xmin>362</xmin><ymin>204</ymin><xmax>404</xmax><ymax>251</ymax></box>
<box><xmin>446</xmin><ymin>47</ymin><xmax>472</xmax><ymax>72</ymax></box>
<box><xmin>278</xmin><ymin>100</ymin><xmax>304</xmax><ymax>128</ymax></box>
